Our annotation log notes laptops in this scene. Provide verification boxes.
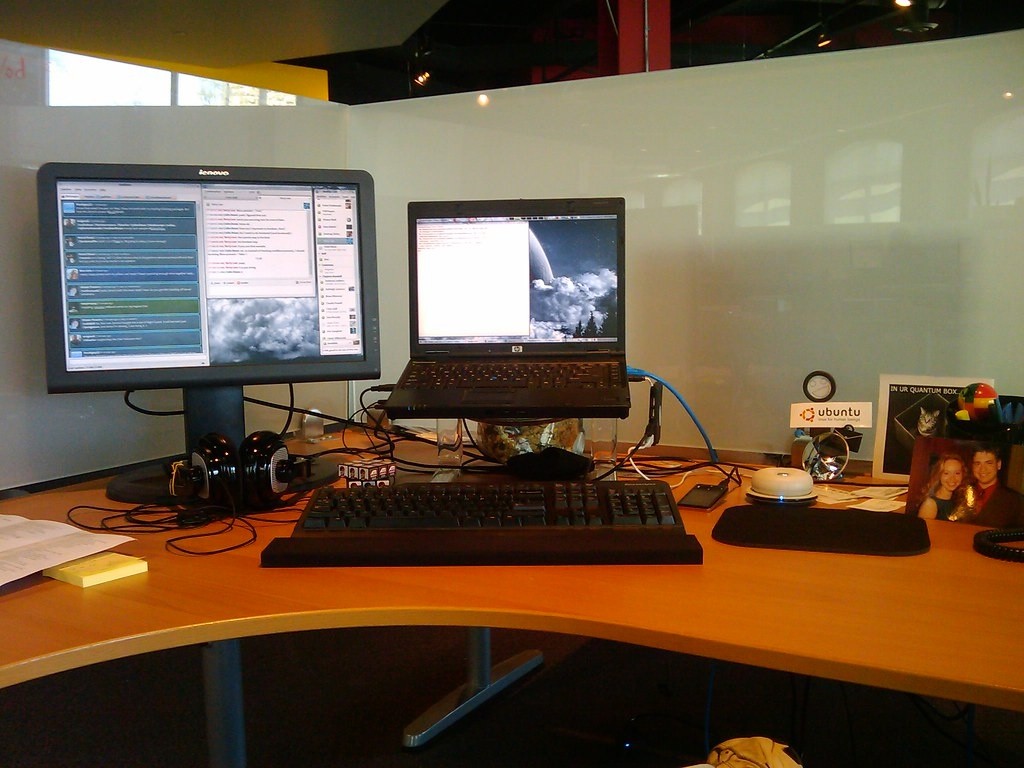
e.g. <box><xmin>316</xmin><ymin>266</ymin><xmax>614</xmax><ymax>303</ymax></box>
<box><xmin>384</xmin><ymin>196</ymin><xmax>630</xmax><ymax>420</ymax></box>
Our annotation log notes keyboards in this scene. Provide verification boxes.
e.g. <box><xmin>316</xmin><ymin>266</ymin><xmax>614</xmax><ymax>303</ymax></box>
<box><xmin>290</xmin><ymin>479</ymin><xmax>689</xmax><ymax>537</ymax></box>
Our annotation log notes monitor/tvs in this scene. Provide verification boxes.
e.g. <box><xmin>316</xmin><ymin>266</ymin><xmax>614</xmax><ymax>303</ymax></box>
<box><xmin>38</xmin><ymin>162</ymin><xmax>380</xmax><ymax>508</ymax></box>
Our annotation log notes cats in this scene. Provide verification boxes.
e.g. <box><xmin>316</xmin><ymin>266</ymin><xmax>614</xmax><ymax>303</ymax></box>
<box><xmin>919</xmin><ymin>407</ymin><xmax>943</xmax><ymax>437</ymax></box>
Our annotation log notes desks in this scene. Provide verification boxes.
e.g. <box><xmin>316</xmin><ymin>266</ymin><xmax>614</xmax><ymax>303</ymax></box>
<box><xmin>0</xmin><ymin>425</ymin><xmax>1024</xmax><ymax>768</ymax></box>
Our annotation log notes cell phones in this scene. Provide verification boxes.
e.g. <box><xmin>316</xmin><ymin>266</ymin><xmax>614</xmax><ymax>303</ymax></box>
<box><xmin>678</xmin><ymin>485</ymin><xmax>727</xmax><ymax>510</ymax></box>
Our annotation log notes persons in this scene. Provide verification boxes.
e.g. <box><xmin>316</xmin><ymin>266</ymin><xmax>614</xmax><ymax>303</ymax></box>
<box><xmin>955</xmin><ymin>442</ymin><xmax>1024</xmax><ymax>527</ymax></box>
<box><xmin>340</xmin><ymin>467</ymin><xmax>388</xmax><ymax>488</ymax></box>
<box><xmin>918</xmin><ymin>454</ymin><xmax>968</xmax><ymax>520</ymax></box>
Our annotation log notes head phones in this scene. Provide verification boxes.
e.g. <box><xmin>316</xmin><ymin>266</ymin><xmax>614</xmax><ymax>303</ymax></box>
<box><xmin>171</xmin><ymin>432</ymin><xmax>313</xmax><ymax>517</ymax></box>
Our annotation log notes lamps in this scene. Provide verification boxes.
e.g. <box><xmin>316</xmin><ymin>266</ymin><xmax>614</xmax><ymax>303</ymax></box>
<box><xmin>404</xmin><ymin>48</ymin><xmax>437</xmax><ymax>89</ymax></box>
